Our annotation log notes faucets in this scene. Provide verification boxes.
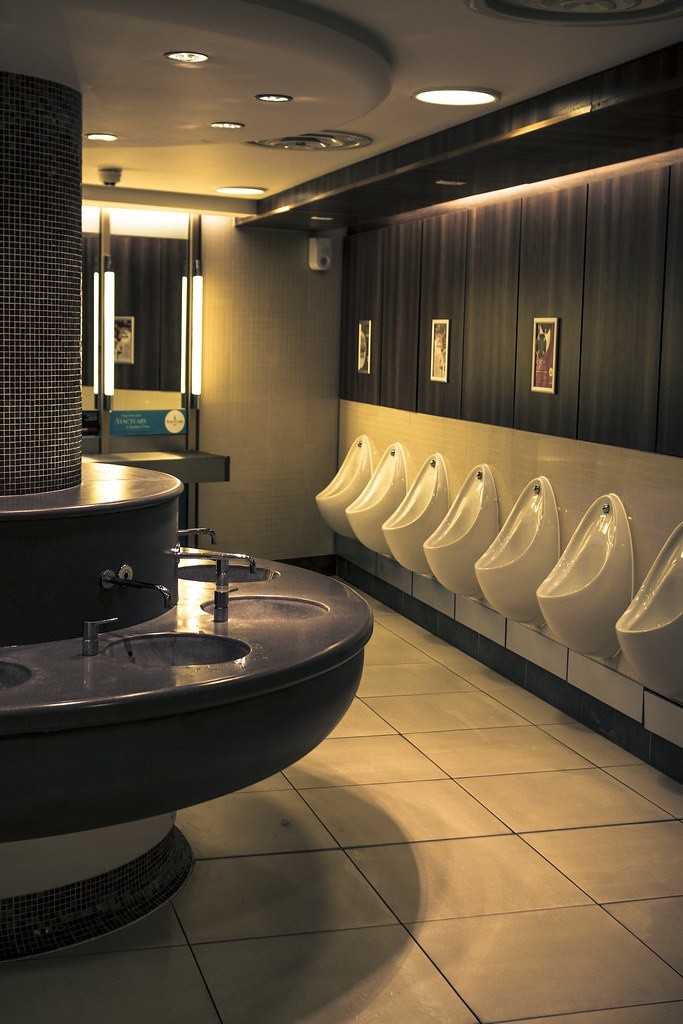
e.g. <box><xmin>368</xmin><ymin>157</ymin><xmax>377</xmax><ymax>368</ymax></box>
<box><xmin>177</xmin><ymin>526</ymin><xmax>216</xmax><ymax>544</ymax></box>
<box><xmin>174</xmin><ymin>542</ymin><xmax>257</xmax><ymax>574</ymax></box>
<box><xmin>100</xmin><ymin>570</ymin><xmax>171</xmax><ymax>610</ymax></box>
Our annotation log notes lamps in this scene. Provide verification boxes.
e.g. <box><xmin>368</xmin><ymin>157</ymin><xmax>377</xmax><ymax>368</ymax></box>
<box><xmin>93</xmin><ymin>255</ymin><xmax>101</xmax><ymax>411</ymax></box>
<box><xmin>104</xmin><ymin>256</ymin><xmax>115</xmax><ymax>413</ymax></box>
<box><xmin>180</xmin><ymin>265</ymin><xmax>187</xmax><ymax>408</ymax></box>
<box><xmin>192</xmin><ymin>261</ymin><xmax>203</xmax><ymax>410</ymax></box>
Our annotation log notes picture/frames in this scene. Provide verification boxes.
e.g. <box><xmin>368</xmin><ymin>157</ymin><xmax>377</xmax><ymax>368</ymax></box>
<box><xmin>115</xmin><ymin>316</ymin><xmax>135</xmax><ymax>365</ymax></box>
<box><xmin>530</xmin><ymin>316</ymin><xmax>560</xmax><ymax>395</ymax></box>
<box><xmin>429</xmin><ymin>318</ymin><xmax>450</xmax><ymax>382</ymax></box>
<box><xmin>357</xmin><ymin>319</ymin><xmax>372</xmax><ymax>375</ymax></box>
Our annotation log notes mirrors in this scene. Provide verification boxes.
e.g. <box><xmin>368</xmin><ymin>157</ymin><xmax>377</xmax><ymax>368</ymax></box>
<box><xmin>104</xmin><ymin>207</ymin><xmax>197</xmax><ymax>451</ymax></box>
<box><xmin>81</xmin><ymin>202</ymin><xmax>102</xmax><ymax>455</ymax></box>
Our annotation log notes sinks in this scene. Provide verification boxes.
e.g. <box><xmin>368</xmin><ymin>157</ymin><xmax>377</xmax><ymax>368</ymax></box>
<box><xmin>0</xmin><ymin>660</ymin><xmax>37</xmax><ymax>689</ymax></box>
<box><xmin>177</xmin><ymin>565</ymin><xmax>281</xmax><ymax>584</ymax></box>
<box><xmin>199</xmin><ymin>594</ymin><xmax>330</xmax><ymax>623</ymax></box>
<box><xmin>105</xmin><ymin>631</ymin><xmax>251</xmax><ymax>668</ymax></box>
